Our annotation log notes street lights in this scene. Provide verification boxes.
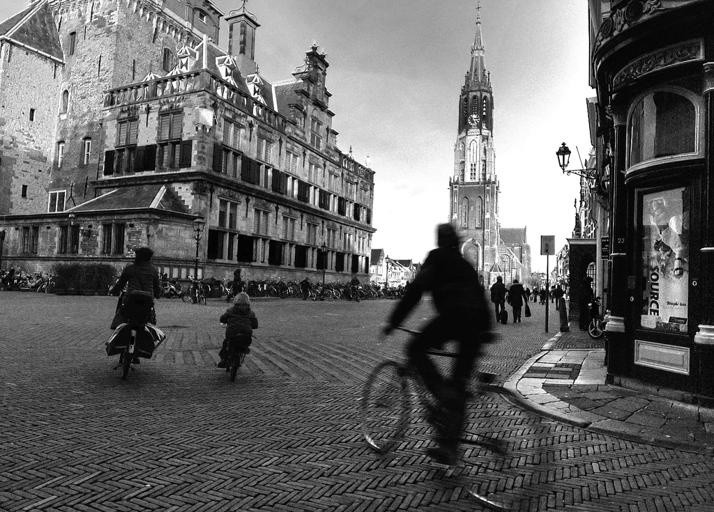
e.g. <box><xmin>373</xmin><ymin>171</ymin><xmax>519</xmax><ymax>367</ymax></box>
<box><xmin>385</xmin><ymin>255</ymin><xmax>390</xmax><ymax>289</ymax></box>
<box><xmin>191</xmin><ymin>218</ymin><xmax>205</xmax><ymax>303</ymax></box>
<box><xmin>320</xmin><ymin>243</ymin><xmax>328</xmax><ymax>301</ymax></box>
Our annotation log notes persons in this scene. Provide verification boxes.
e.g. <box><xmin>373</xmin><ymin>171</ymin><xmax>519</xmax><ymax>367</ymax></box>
<box><xmin>224</xmin><ymin>268</ymin><xmax>245</xmax><ymax>302</ymax></box>
<box><xmin>646</xmin><ymin>195</ymin><xmax>688</xmax><ymax>279</ymax></box>
<box><xmin>108</xmin><ymin>246</ymin><xmax>161</xmax><ymax>327</ymax></box>
<box><xmin>349</xmin><ymin>275</ymin><xmax>361</xmax><ymax>296</ymax></box>
<box><xmin>216</xmin><ymin>291</ymin><xmax>258</xmax><ymax>369</ymax></box>
<box><xmin>299</xmin><ymin>276</ymin><xmax>315</xmax><ymax>300</ymax></box>
<box><xmin>380</xmin><ymin>222</ymin><xmax>494</xmax><ymax>465</ymax></box>
<box><xmin>478</xmin><ymin>273</ymin><xmax>597</xmax><ymax>331</ymax></box>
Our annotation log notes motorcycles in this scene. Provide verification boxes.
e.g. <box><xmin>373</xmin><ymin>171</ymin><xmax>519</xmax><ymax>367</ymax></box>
<box><xmin>587</xmin><ymin>294</ymin><xmax>605</xmax><ymax>339</ymax></box>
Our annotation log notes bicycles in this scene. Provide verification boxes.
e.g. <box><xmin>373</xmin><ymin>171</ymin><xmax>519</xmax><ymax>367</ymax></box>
<box><xmin>159</xmin><ymin>272</ymin><xmax>406</xmax><ymax>305</ymax></box>
<box><xmin>16</xmin><ymin>271</ymin><xmax>53</xmax><ymax>293</ymax></box>
<box><xmin>219</xmin><ymin>316</ymin><xmax>253</xmax><ymax>381</ymax></box>
<box><xmin>360</xmin><ymin>324</ymin><xmax>552</xmax><ymax>512</ymax></box>
<box><xmin>107</xmin><ymin>282</ymin><xmax>156</xmax><ymax>381</ymax></box>
